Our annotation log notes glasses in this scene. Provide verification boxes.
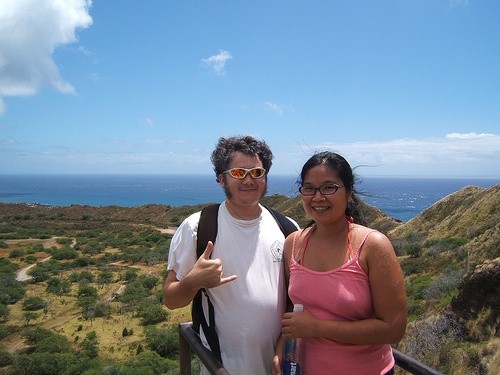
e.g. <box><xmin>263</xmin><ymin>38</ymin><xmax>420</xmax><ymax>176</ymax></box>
<box><xmin>299</xmin><ymin>184</ymin><xmax>345</xmax><ymax>195</ymax></box>
<box><xmin>222</xmin><ymin>167</ymin><xmax>267</xmax><ymax>180</ymax></box>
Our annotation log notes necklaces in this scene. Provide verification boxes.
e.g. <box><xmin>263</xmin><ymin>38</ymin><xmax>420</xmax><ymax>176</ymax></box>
<box><xmin>226</xmin><ymin>202</ymin><xmax>260</xmax><ymax>221</ymax></box>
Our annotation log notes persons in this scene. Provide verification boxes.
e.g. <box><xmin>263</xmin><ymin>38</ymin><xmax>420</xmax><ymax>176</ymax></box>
<box><xmin>162</xmin><ymin>135</ymin><xmax>302</xmax><ymax>375</ymax></box>
<box><xmin>271</xmin><ymin>151</ymin><xmax>408</xmax><ymax>375</ymax></box>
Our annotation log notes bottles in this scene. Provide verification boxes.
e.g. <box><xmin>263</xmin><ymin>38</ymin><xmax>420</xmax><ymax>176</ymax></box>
<box><xmin>282</xmin><ymin>304</ymin><xmax>303</xmax><ymax>375</ymax></box>
